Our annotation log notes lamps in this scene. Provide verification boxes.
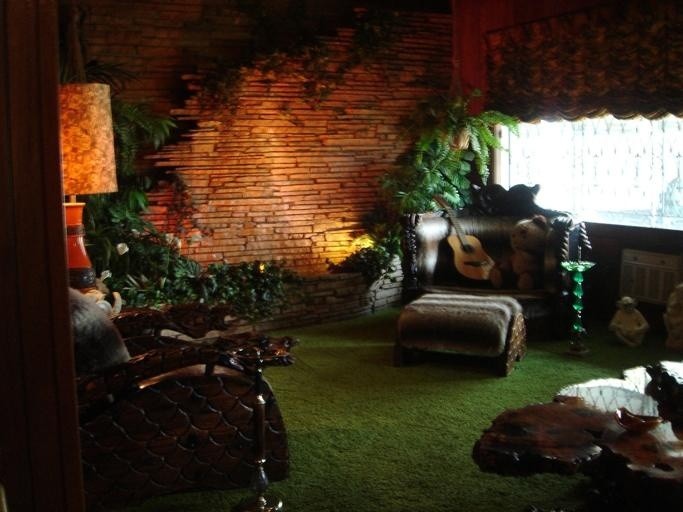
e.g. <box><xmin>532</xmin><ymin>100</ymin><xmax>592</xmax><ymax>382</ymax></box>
<box><xmin>57</xmin><ymin>82</ymin><xmax>119</xmax><ymax>286</ymax></box>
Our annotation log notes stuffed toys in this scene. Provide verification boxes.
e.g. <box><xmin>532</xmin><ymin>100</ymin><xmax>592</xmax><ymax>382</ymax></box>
<box><xmin>489</xmin><ymin>215</ymin><xmax>548</xmax><ymax>290</ymax></box>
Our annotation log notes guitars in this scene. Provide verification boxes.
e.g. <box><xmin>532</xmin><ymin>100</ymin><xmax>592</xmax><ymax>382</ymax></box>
<box><xmin>432</xmin><ymin>194</ymin><xmax>494</xmax><ymax>287</ymax></box>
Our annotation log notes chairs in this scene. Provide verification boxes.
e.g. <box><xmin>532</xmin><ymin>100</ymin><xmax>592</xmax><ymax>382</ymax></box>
<box><xmin>67</xmin><ymin>287</ymin><xmax>301</xmax><ymax>512</ymax></box>
<box><xmin>401</xmin><ymin>184</ymin><xmax>570</xmax><ymax>334</ymax></box>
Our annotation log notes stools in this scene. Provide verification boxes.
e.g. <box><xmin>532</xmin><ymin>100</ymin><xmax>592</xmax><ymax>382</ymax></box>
<box><xmin>392</xmin><ymin>290</ymin><xmax>528</xmax><ymax>377</ymax></box>
